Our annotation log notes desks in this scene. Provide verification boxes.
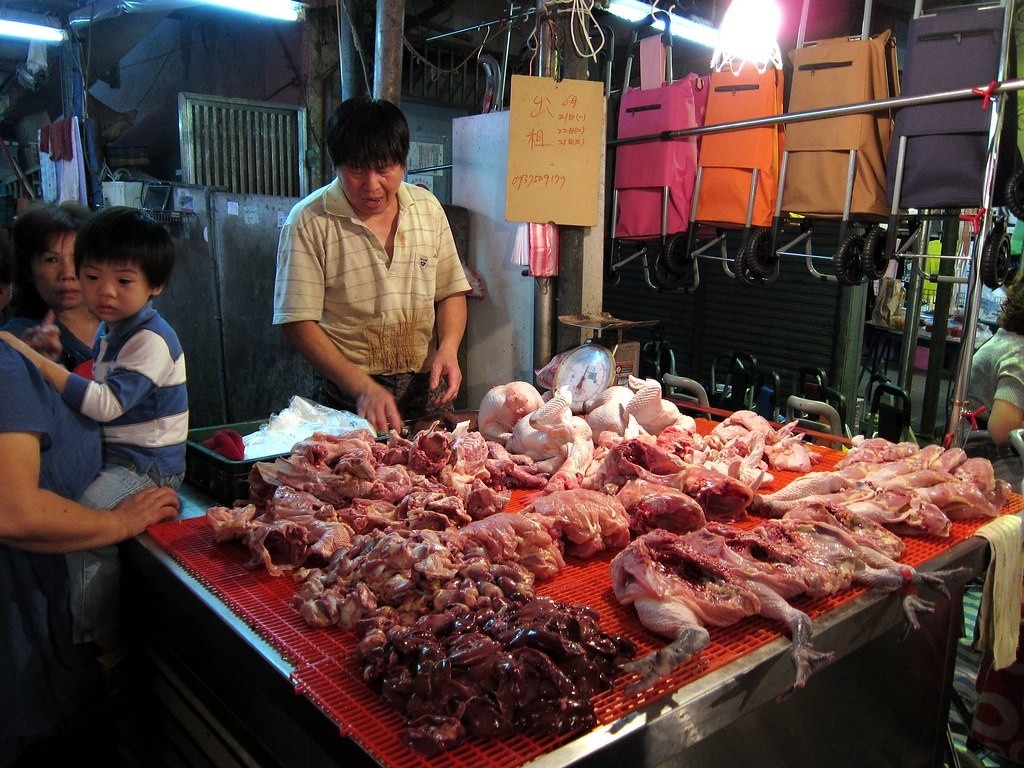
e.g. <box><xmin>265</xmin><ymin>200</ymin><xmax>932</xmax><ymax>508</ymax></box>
<box><xmin>858</xmin><ymin>320</ymin><xmax>961</xmax><ymax>393</ymax></box>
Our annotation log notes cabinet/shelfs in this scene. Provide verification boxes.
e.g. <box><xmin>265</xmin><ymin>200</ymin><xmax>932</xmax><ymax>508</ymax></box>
<box><xmin>102</xmin><ymin>182</ymin><xmax>314</xmax><ymax>430</ymax></box>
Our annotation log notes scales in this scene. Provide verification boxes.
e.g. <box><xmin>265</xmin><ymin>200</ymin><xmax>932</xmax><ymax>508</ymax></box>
<box><xmin>551</xmin><ymin>311</ymin><xmax>660</xmax><ymax>413</ymax></box>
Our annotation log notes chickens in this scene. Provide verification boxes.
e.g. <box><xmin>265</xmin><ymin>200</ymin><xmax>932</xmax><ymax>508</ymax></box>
<box><xmin>605</xmin><ymin>499</ymin><xmax>977</xmax><ymax>697</ymax></box>
<box><xmin>580</xmin><ymin>373</ymin><xmax>698</xmax><ymax>447</ymax></box>
<box><xmin>494</xmin><ymin>386</ymin><xmax>591</xmax><ymax>498</ymax></box>
<box><xmin>477</xmin><ymin>381</ymin><xmax>544</xmax><ymax>445</ymax></box>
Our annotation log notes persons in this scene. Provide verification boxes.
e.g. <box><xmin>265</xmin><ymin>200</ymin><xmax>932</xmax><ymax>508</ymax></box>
<box><xmin>964</xmin><ymin>274</ymin><xmax>1024</xmax><ymax>496</ymax></box>
<box><xmin>0</xmin><ymin>203</ymin><xmax>189</xmax><ymax>768</ymax></box>
<box><xmin>272</xmin><ymin>98</ymin><xmax>474</xmax><ymax>437</ymax></box>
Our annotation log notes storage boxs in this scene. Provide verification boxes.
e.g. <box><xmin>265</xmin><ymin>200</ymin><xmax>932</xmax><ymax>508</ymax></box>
<box><xmin>185</xmin><ymin>419</ymin><xmax>293</xmax><ymax>507</ymax></box>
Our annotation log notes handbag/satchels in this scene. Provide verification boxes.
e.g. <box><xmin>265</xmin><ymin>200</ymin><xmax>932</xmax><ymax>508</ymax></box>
<box><xmin>614</xmin><ymin>11</ymin><xmax>710</xmax><ymax>242</ymax></box>
<box><xmin>693</xmin><ymin>63</ymin><xmax>787</xmax><ymax>230</ymax></box>
<box><xmin>890</xmin><ymin>1</ymin><xmax>1021</xmax><ymax>205</ymax></box>
<box><xmin>785</xmin><ymin>1</ymin><xmax>899</xmax><ymax>216</ymax></box>
